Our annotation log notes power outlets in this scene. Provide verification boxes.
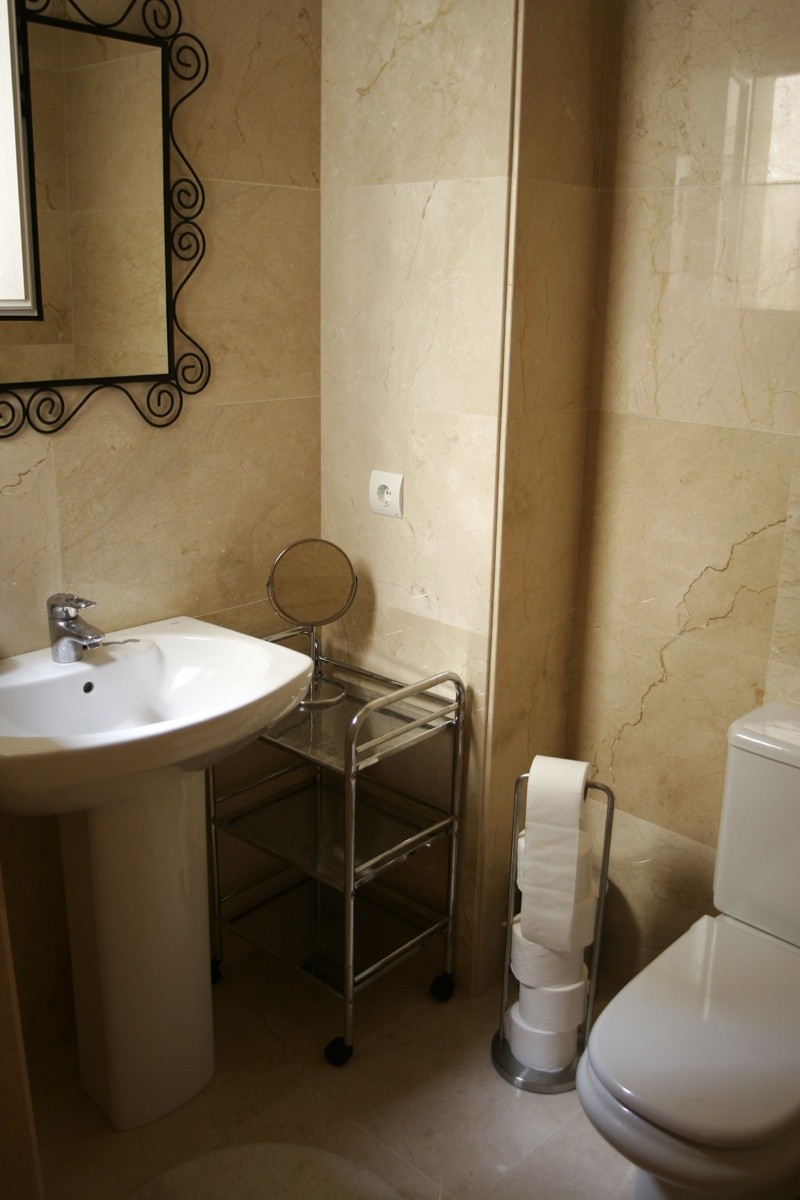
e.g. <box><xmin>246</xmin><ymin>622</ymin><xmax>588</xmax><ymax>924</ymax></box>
<box><xmin>369</xmin><ymin>470</ymin><xmax>404</xmax><ymax>519</ymax></box>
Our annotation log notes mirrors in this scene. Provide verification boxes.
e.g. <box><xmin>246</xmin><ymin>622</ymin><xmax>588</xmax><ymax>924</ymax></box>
<box><xmin>0</xmin><ymin>0</ymin><xmax>211</xmax><ymax>438</ymax></box>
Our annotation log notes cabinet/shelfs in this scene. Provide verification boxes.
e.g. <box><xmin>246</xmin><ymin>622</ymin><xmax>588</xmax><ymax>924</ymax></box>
<box><xmin>207</xmin><ymin>626</ymin><xmax>467</xmax><ymax>1069</ymax></box>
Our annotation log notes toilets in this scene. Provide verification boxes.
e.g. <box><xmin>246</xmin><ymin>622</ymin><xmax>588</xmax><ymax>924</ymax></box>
<box><xmin>574</xmin><ymin>701</ymin><xmax>799</xmax><ymax>1200</ymax></box>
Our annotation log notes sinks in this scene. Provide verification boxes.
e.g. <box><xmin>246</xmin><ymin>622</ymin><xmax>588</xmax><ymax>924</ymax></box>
<box><xmin>0</xmin><ymin>616</ymin><xmax>316</xmax><ymax>814</ymax></box>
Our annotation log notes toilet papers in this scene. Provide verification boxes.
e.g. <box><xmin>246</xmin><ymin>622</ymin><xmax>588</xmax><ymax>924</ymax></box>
<box><xmin>501</xmin><ymin>754</ymin><xmax>609</xmax><ymax>1073</ymax></box>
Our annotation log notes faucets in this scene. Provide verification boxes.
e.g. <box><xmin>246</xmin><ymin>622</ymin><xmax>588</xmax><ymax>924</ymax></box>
<box><xmin>46</xmin><ymin>593</ymin><xmax>105</xmax><ymax>663</ymax></box>
